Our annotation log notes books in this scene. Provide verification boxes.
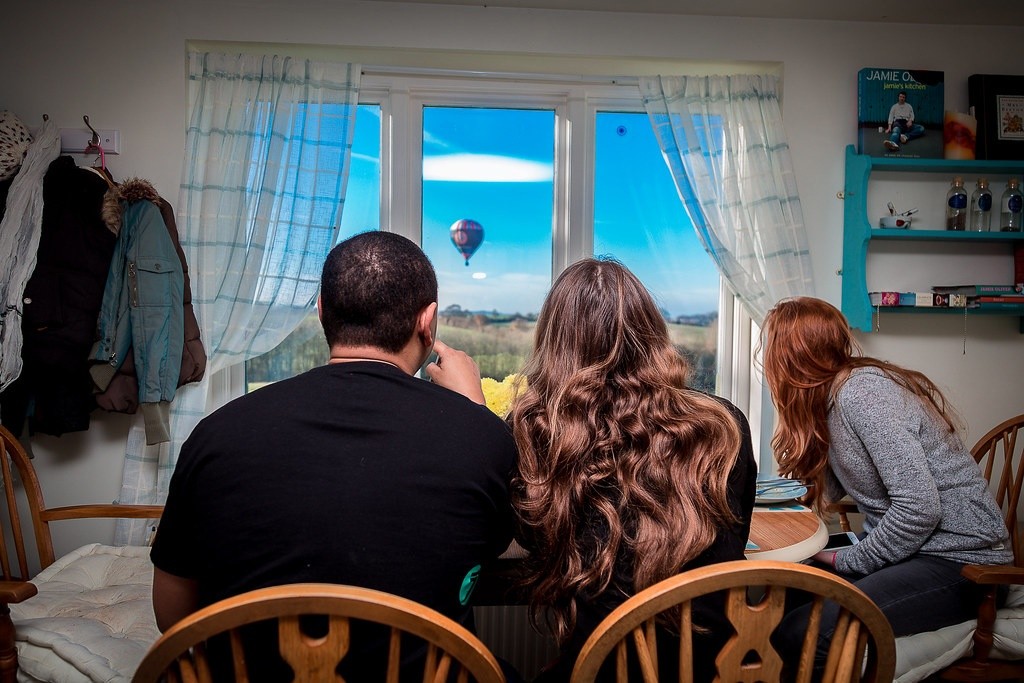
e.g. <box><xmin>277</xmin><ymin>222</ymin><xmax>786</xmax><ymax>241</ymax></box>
<box><xmin>968</xmin><ymin>74</ymin><xmax>1024</xmax><ymax>161</ymax></box>
<box><xmin>931</xmin><ymin>285</ymin><xmax>1024</xmax><ymax>310</ymax></box>
<box><xmin>868</xmin><ymin>292</ymin><xmax>967</xmax><ymax>307</ymax></box>
<box><xmin>858</xmin><ymin>68</ymin><xmax>945</xmax><ymax>158</ymax></box>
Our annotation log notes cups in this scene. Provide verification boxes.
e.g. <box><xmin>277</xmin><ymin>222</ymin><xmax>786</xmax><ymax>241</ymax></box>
<box><xmin>944</xmin><ymin>110</ymin><xmax>977</xmax><ymax>160</ymax></box>
<box><xmin>879</xmin><ymin>127</ymin><xmax>882</xmax><ymax>133</ymax></box>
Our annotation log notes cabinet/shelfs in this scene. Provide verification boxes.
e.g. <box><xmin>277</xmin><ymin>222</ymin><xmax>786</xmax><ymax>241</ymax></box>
<box><xmin>839</xmin><ymin>142</ymin><xmax>1023</xmax><ymax>335</ymax></box>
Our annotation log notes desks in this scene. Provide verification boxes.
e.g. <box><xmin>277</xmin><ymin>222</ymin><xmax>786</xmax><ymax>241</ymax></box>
<box><xmin>475</xmin><ymin>499</ymin><xmax>832</xmax><ymax>605</ymax></box>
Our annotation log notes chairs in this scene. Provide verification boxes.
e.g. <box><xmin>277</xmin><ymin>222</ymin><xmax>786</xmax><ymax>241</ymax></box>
<box><xmin>568</xmin><ymin>557</ymin><xmax>898</xmax><ymax>682</ymax></box>
<box><xmin>129</xmin><ymin>582</ymin><xmax>506</xmax><ymax>683</ymax></box>
<box><xmin>926</xmin><ymin>415</ymin><xmax>1024</xmax><ymax>683</ymax></box>
<box><xmin>0</xmin><ymin>426</ymin><xmax>168</xmax><ymax>683</ymax></box>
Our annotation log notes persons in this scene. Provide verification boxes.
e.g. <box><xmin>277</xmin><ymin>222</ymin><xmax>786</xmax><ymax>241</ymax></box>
<box><xmin>151</xmin><ymin>232</ymin><xmax>520</xmax><ymax>683</ymax></box>
<box><xmin>883</xmin><ymin>91</ymin><xmax>925</xmax><ymax>151</ymax></box>
<box><xmin>755</xmin><ymin>296</ymin><xmax>1012</xmax><ymax>683</ymax></box>
<box><xmin>503</xmin><ymin>257</ymin><xmax>758</xmax><ymax>683</ymax></box>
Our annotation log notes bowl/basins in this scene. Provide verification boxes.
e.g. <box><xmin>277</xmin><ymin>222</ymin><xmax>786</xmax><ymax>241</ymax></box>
<box><xmin>879</xmin><ymin>216</ymin><xmax>913</xmax><ymax>229</ymax></box>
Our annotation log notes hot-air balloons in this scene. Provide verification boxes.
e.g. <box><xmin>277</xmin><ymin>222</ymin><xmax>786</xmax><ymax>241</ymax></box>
<box><xmin>449</xmin><ymin>218</ymin><xmax>485</xmax><ymax>266</ymax></box>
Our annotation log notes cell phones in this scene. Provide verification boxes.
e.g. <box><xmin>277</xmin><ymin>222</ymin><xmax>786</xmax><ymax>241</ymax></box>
<box><xmin>821</xmin><ymin>531</ymin><xmax>858</xmax><ymax>552</ymax></box>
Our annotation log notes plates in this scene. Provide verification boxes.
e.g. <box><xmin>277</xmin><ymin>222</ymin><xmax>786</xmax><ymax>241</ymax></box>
<box><xmin>754</xmin><ymin>480</ymin><xmax>807</xmax><ymax>505</ymax></box>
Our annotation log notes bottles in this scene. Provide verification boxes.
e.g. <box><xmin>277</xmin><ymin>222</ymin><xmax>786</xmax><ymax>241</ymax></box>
<box><xmin>1000</xmin><ymin>179</ymin><xmax>1024</xmax><ymax>233</ymax></box>
<box><xmin>945</xmin><ymin>175</ymin><xmax>968</xmax><ymax>230</ymax></box>
<box><xmin>969</xmin><ymin>177</ymin><xmax>993</xmax><ymax>232</ymax></box>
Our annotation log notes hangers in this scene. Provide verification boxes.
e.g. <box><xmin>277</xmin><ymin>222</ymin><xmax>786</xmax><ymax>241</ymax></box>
<box><xmin>84</xmin><ymin>144</ymin><xmax>105</xmax><ymax>170</ymax></box>
